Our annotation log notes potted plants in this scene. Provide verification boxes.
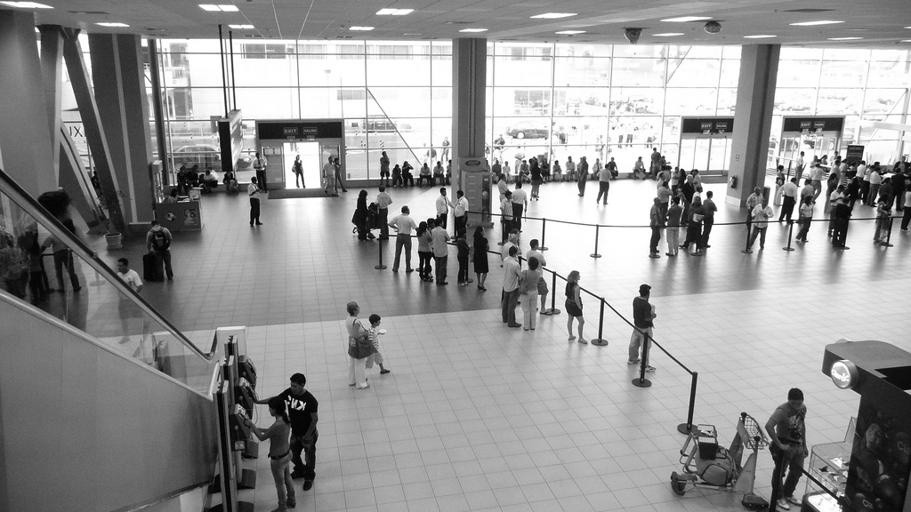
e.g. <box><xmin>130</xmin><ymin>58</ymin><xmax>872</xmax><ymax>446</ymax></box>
<box><xmin>98</xmin><ymin>189</ymin><xmax>135</xmax><ymax>250</ymax></box>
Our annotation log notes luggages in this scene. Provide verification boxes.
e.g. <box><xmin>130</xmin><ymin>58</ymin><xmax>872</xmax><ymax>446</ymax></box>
<box><xmin>142</xmin><ymin>250</ymin><xmax>164</xmax><ymax>282</ymax></box>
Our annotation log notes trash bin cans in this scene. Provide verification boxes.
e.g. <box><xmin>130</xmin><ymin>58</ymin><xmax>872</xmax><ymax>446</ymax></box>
<box><xmin>762</xmin><ymin>186</ymin><xmax>770</xmax><ymax>209</ymax></box>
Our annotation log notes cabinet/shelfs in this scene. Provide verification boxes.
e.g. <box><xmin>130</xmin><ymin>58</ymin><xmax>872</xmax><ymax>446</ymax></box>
<box><xmin>800</xmin><ymin>417</ymin><xmax>860</xmax><ymax>512</ymax></box>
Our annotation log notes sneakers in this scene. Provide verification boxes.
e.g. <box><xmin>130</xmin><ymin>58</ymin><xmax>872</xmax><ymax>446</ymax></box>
<box><xmin>640</xmin><ymin>365</ymin><xmax>656</xmax><ymax>372</ymax></box>
<box><xmin>289</xmin><ymin>470</ymin><xmax>303</xmax><ymax>479</ymax></box>
<box><xmin>302</xmin><ymin>479</ymin><xmax>311</xmax><ymax>490</ymax></box>
<box><xmin>380</xmin><ymin>369</ymin><xmax>390</xmax><ymax>374</ymax></box>
<box><xmin>578</xmin><ymin>338</ymin><xmax>587</xmax><ymax>344</ymax></box>
<box><xmin>392</xmin><ymin>269</ymin><xmax>487</xmax><ymax>291</ymax></box>
<box><xmin>783</xmin><ymin>494</ymin><xmax>803</xmax><ymax>506</ymax></box>
<box><xmin>776</xmin><ymin>496</ymin><xmax>790</xmax><ymax>510</ymax></box>
<box><xmin>508</xmin><ymin>323</ymin><xmax>521</xmax><ymax>327</ymax></box>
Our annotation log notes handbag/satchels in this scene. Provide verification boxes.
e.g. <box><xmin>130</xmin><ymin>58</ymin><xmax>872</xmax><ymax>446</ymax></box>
<box><xmin>769</xmin><ymin>437</ymin><xmax>785</xmax><ymax>462</ymax></box>
<box><xmin>348</xmin><ymin>319</ymin><xmax>372</xmax><ymax>359</ymax></box>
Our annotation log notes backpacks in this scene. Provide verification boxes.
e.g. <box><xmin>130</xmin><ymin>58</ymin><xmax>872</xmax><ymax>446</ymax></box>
<box><xmin>149</xmin><ymin>226</ymin><xmax>170</xmax><ymax>252</ymax></box>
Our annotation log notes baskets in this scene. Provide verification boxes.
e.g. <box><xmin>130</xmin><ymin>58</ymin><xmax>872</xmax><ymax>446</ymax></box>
<box><xmin>736</xmin><ymin>414</ymin><xmax>768</xmax><ymax>449</ymax></box>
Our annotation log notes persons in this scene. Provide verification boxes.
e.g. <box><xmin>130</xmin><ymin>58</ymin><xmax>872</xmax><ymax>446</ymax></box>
<box><xmin>354</xmin><ymin>184</ymin><xmax>469</xmax><ymax>285</ymax></box>
<box><xmin>378</xmin><ymin>137</ymin><xmax>452</xmax><ymax>189</ymax></box>
<box><xmin>486</xmin><ymin>154</ymin><xmax>617</xmax><ymax>239</ymax></box>
<box><xmin>176</xmin><ymin>151</ymin><xmax>268</xmax><ymax>227</ymax></box>
<box><xmin>633</xmin><ymin>147</ymin><xmax>717</xmax><ymax>259</ymax></box>
<box><xmin>766</xmin><ymin>388</ymin><xmax>808</xmax><ymax>510</ymax></box>
<box><xmin>345</xmin><ymin>301</ymin><xmax>390</xmax><ymax>389</ymax></box>
<box><xmin>743</xmin><ymin>152</ymin><xmax>911</xmax><ymax>247</ymax></box>
<box><xmin>244</xmin><ymin>398</ymin><xmax>294</xmax><ymax>512</ymax></box>
<box><xmin>627</xmin><ymin>283</ymin><xmax>658</xmax><ymax>372</ymax></box>
<box><xmin>294</xmin><ymin>154</ymin><xmax>348</xmax><ymax>194</ymax></box>
<box><xmin>472</xmin><ymin>224</ymin><xmax>589</xmax><ymax>345</ymax></box>
<box><xmin>1</xmin><ymin>207</ymin><xmax>173</xmax><ymax>343</ymax></box>
<box><xmin>253</xmin><ymin>373</ymin><xmax>319</xmax><ymax>489</ymax></box>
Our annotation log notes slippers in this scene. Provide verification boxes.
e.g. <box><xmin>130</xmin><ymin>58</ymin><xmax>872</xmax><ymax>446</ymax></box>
<box><xmin>540</xmin><ymin>311</ymin><xmax>553</xmax><ymax>315</ymax></box>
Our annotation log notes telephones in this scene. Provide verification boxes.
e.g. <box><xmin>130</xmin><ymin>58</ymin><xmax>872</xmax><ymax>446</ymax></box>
<box><xmin>230</xmin><ymin>355</ymin><xmax>256</xmax><ymax>442</ymax></box>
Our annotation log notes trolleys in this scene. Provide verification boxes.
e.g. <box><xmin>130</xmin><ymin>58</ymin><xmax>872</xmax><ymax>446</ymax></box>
<box><xmin>669</xmin><ymin>409</ymin><xmax>770</xmax><ymax>497</ymax></box>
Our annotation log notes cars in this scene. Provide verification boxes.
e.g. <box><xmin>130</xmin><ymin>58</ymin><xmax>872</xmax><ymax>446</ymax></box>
<box><xmin>161</xmin><ymin>143</ymin><xmax>252</xmax><ymax>171</ymax></box>
<box><xmin>766</xmin><ymin>136</ymin><xmax>860</xmax><ymax>168</ymax></box>
<box><xmin>504</xmin><ymin>123</ymin><xmax>555</xmax><ymax>138</ymax></box>
<box><xmin>346</xmin><ymin>112</ymin><xmax>412</xmax><ymax>133</ymax></box>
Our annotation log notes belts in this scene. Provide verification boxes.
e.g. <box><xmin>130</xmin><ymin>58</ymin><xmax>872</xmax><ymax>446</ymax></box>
<box><xmin>267</xmin><ymin>447</ymin><xmax>290</xmax><ymax>460</ymax></box>
